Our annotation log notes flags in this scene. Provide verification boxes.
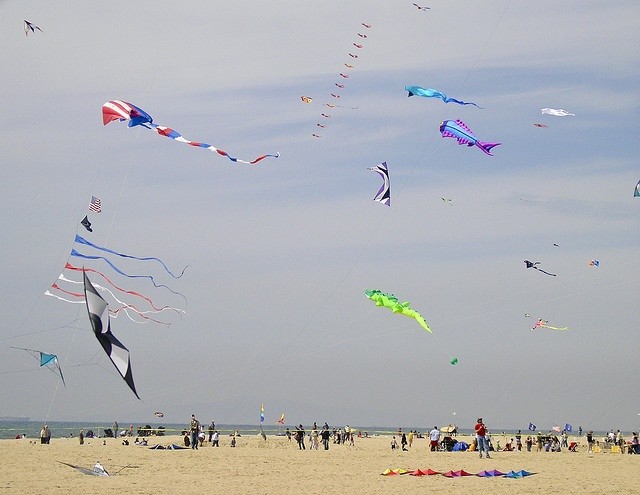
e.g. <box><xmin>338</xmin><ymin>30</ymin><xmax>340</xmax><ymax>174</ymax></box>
<box><xmin>552</xmin><ymin>425</ymin><xmax>561</xmax><ymax>433</ymax></box>
<box><xmin>79</xmin><ymin>214</ymin><xmax>93</xmax><ymax>233</ymax></box>
<box><xmin>528</xmin><ymin>422</ymin><xmax>537</xmax><ymax>432</ymax></box>
<box><xmin>565</xmin><ymin>422</ymin><xmax>573</xmax><ymax>432</ymax></box>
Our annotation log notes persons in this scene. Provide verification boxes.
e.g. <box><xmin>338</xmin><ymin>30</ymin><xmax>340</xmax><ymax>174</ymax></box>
<box><xmin>614</xmin><ymin>429</ymin><xmax>621</xmax><ymax>444</ymax></box>
<box><xmin>417</xmin><ymin>432</ymin><xmax>421</xmax><ymax>437</ymax></box>
<box><xmin>535</xmin><ymin>435</ymin><xmax>542</xmax><ymax>452</ymax></box>
<box><xmin>503</xmin><ymin>438</ymin><xmax>514</xmax><ymax>451</ymax></box>
<box><xmin>16</xmin><ymin>434</ymin><xmax>20</xmax><ymax>439</ymax></box>
<box><xmin>334</xmin><ymin>432</ymin><xmax>341</xmax><ymax>444</ymax></box>
<box><xmin>532</xmin><ymin>437</ymin><xmax>535</xmax><ymax>445</ymax></box>
<box><xmin>323</xmin><ymin>422</ymin><xmax>329</xmax><ymax>430</ymax></box>
<box><xmin>474</xmin><ymin>417</ymin><xmax>491</xmax><ymax>459</ymax></box>
<box><xmin>358</xmin><ymin>431</ymin><xmax>361</xmax><ymax>437</ymax></box>
<box><xmin>133</xmin><ymin>437</ymin><xmax>140</xmax><ymax>444</ymax></box>
<box><xmin>568</xmin><ymin>442</ymin><xmax>580</xmax><ymax>452</ymax></box>
<box><xmin>126</xmin><ymin>440</ymin><xmax>129</xmax><ymax>445</ymax></box>
<box><xmin>141</xmin><ymin>438</ymin><xmax>147</xmax><ymax>445</ymax></box>
<box><xmin>112</xmin><ymin>420</ymin><xmax>118</xmax><ymax>438</ymax></box>
<box><xmin>294</xmin><ymin>424</ymin><xmax>306</xmax><ymax>450</ymax></box>
<box><xmin>447</xmin><ymin>424</ymin><xmax>452</xmax><ymax>436</ymax></box>
<box><xmin>595</xmin><ymin>441</ymin><xmax>601</xmax><ymax>453</ymax></box>
<box><xmin>560</xmin><ymin>430</ymin><xmax>565</xmax><ymax>448</ymax></box>
<box><xmin>631</xmin><ymin>431</ymin><xmax>639</xmax><ymax>445</ymax></box>
<box><xmin>390</xmin><ymin>436</ymin><xmax>397</xmax><ymax>449</ymax></box>
<box><xmin>494</xmin><ymin>440</ymin><xmax>502</xmax><ymax>452</ymax></box>
<box><xmin>40</xmin><ymin>427</ymin><xmax>46</xmax><ymax>438</ymax></box>
<box><xmin>563</xmin><ymin>429</ymin><xmax>569</xmax><ymax>447</ymax></box>
<box><xmin>333</xmin><ymin>428</ymin><xmax>338</xmax><ymax>439</ymax></box>
<box><xmin>611</xmin><ymin>442</ymin><xmax>621</xmax><ymax>453</ymax></box>
<box><xmin>208</xmin><ymin>420</ymin><xmax>216</xmax><ymax>442</ymax></box>
<box><xmin>609</xmin><ymin>429</ymin><xmax>615</xmax><ymax>440</ymax></box>
<box><xmin>364</xmin><ymin>432</ymin><xmax>368</xmax><ymax>438</ymax></box>
<box><xmin>313</xmin><ymin>422</ymin><xmax>317</xmax><ymax>430</ymax></box>
<box><xmin>346</xmin><ymin>426</ymin><xmax>350</xmax><ymax>441</ymax></box>
<box><xmin>592</xmin><ymin>439</ymin><xmax>595</xmax><ymax>453</ymax></box>
<box><xmin>130</xmin><ymin>424</ymin><xmax>134</xmax><ymax>437</ymax></box>
<box><xmin>79</xmin><ymin>428</ymin><xmax>84</xmax><ymax>445</ymax></box>
<box><xmin>578</xmin><ymin>425</ymin><xmax>582</xmax><ymax>437</ymax></box>
<box><xmin>191</xmin><ymin>420</ymin><xmax>200</xmax><ymax>450</ymax></box>
<box><xmin>401</xmin><ymin>433</ymin><xmax>408</xmax><ymax>449</ymax></box>
<box><xmin>137</xmin><ymin>424</ymin><xmax>165</xmax><ymax>436</ymax></box>
<box><xmin>350</xmin><ymin>433</ymin><xmax>355</xmax><ymax>447</ymax></box>
<box><xmin>260</xmin><ymin>429</ymin><xmax>267</xmax><ymax>441</ymax></box>
<box><xmin>338</xmin><ymin>428</ymin><xmax>341</xmax><ymax>432</ymax></box>
<box><xmin>308</xmin><ymin>431</ymin><xmax>319</xmax><ymax>450</ymax></box>
<box><xmin>212</xmin><ymin>431</ymin><xmax>220</xmax><ymax>447</ymax></box>
<box><xmin>122</xmin><ymin>440</ymin><xmax>126</xmax><ymax>445</ymax></box>
<box><xmin>22</xmin><ymin>434</ymin><xmax>26</xmax><ymax>439</ymax></box>
<box><xmin>322</xmin><ymin>426</ymin><xmax>329</xmax><ymax>450</ymax></box>
<box><xmin>466</xmin><ymin>439</ymin><xmax>475</xmax><ymax>451</ymax></box>
<box><xmin>587</xmin><ymin>431</ymin><xmax>593</xmax><ymax>453</ymax></box>
<box><xmin>344</xmin><ymin>425</ymin><xmax>348</xmax><ymax>431</ymax></box>
<box><xmin>45</xmin><ymin>425</ymin><xmax>51</xmax><ymax>444</ymax></box>
<box><xmin>398</xmin><ymin>427</ymin><xmax>402</xmax><ymax>437</ymax></box>
<box><xmin>604</xmin><ymin>432</ymin><xmax>611</xmax><ymax>442</ymax></box>
<box><xmin>516</xmin><ymin>429</ymin><xmax>522</xmax><ymax>440</ymax></box>
<box><xmin>230</xmin><ymin>437</ymin><xmax>236</xmax><ymax>447</ymax></box>
<box><xmin>190</xmin><ymin>414</ymin><xmax>198</xmax><ymax>421</ymax></box>
<box><xmin>119</xmin><ymin>429</ymin><xmax>128</xmax><ymax>437</ymax></box>
<box><xmin>183</xmin><ymin>432</ymin><xmax>190</xmax><ymax>446</ymax></box>
<box><xmin>516</xmin><ymin>438</ymin><xmax>522</xmax><ymax>451</ymax></box>
<box><xmin>341</xmin><ymin>431</ymin><xmax>346</xmax><ymax>444</ymax></box>
<box><xmin>487</xmin><ymin>438</ymin><xmax>494</xmax><ymax>451</ymax></box>
<box><xmin>408</xmin><ymin>430</ymin><xmax>417</xmax><ymax>447</ymax></box>
<box><xmin>544</xmin><ymin>430</ymin><xmax>560</xmax><ymax>452</ymax></box>
<box><xmin>525</xmin><ymin>437</ymin><xmax>532</xmax><ymax>452</ymax></box>
<box><xmin>429</xmin><ymin>425</ymin><xmax>440</xmax><ymax>446</ymax></box>
<box><xmin>453</xmin><ymin>424</ymin><xmax>459</xmax><ymax>437</ymax></box>
<box><xmin>198</xmin><ymin>432</ymin><xmax>206</xmax><ymax>447</ymax></box>
<box><xmin>286</xmin><ymin>427</ymin><xmax>291</xmax><ymax>442</ymax></box>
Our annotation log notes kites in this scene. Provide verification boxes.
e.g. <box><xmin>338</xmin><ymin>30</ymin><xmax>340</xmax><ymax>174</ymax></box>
<box><xmin>68</xmin><ymin>248</ymin><xmax>187</xmax><ymax>306</ymax></box>
<box><xmin>541</xmin><ymin>108</ymin><xmax>576</xmax><ymax>118</ymax></box>
<box><xmin>42</xmin><ymin>289</ymin><xmax>149</xmax><ymax>326</ymax></box>
<box><xmin>366</xmin><ymin>160</ymin><xmax>391</xmax><ymax>208</ymax></box>
<box><xmin>531</xmin><ymin>317</ymin><xmax>568</xmax><ymax>332</ymax></box>
<box><xmin>297</xmin><ymin>94</ymin><xmax>313</xmax><ymax>104</ymax></box>
<box><xmin>353</xmin><ymin>42</ymin><xmax>365</xmax><ymax>48</ymax></box>
<box><xmin>22</xmin><ymin>18</ymin><xmax>46</xmax><ymax>36</ymax></box>
<box><xmin>502</xmin><ymin>469</ymin><xmax>537</xmax><ymax>477</ymax></box>
<box><xmin>408</xmin><ymin>468</ymin><xmax>441</xmax><ymax>475</ymax></box>
<box><xmin>347</xmin><ymin>52</ymin><xmax>359</xmax><ymax>59</ymax></box>
<box><xmin>441</xmin><ymin>470</ymin><xmax>475</xmax><ymax>477</ymax></box>
<box><xmin>363</xmin><ymin>287</ymin><xmax>434</xmax><ymax>335</ymax></box>
<box><xmin>72</xmin><ymin>234</ymin><xmax>191</xmax><ymax>279</ymax></box>
<box><xmin>344</xmin><ymin>62</ymin><xmax>354</xmax><ymax>68</ymax></box>
<box><xmin>88</xmin><ymin>194</ymin><xmax>104</xmax><ymax>214</ymax></box>
<box><xmin>530</xmin><ymin>122</ymin><xmax>549</xmax><ymax>128</ymax></box>
<box><xmin>404</xmin><ymin>85</ymin><xmax>485</xmax><ymax>110</ymax></box>
<box><xmin>634</xmin><ymin>179</ymin><xmax>640</xmax><ymax>198</ymax></box>
<box><xmin>326</xmin><ymin>102</ymin><xmax>336</xmax><ymax>108</ymax></box>
<box><xmin>62</xmin><ymin>260</ymin><xmax>183</xmax><ymax>319</ymax></box>
<box><xmin>588</xmin><ymin>259</ymin><xmax>599</xmax><ymax>269</ymax></box>
<box><xmin>452</xmin><ymin>357</ymin><xmax>461</xmax><ymax>367</ymax></box>
<box><xmin>48</xmin><ymin>282</ymin><xmax>171</xmax><ymax>326</ymax></box>
<box><xmin>56</xmin><ymin>272</ymin><xmax>189</xmax><ymax>317</ymax></box>
<box><xmin>334</xmin><ymin>82</ymin><xmax>345</xmax><ymax>89</ymax></box>
<box><xmin>322</xmin><ymin>112</ymin><xmax>331</xmax><ymax>119</ymax></box>
<box><xmin>361</xmin><ymin>23</ymin><xmax>371</xmax><ymax>29</ymax></box>
<box><xmin>58</xmin><ymin>460</ymin><xmax>110</xmax><ymax>476</ymax></box>
<box><xmin>523</xmin><ymin>259</ymin><xmax>557</xmax><ymax>277</ymax></box>
<box><xmin>82</xmin><ymin>266</ymin><xmax>142</xmax><ymax>402</ymax></box>
<box><xmin>439</xmin><ymin>117</ymin><xmax>502</xmax><ymax>157</ymax></box>
<box><xmin>316</xmin><ymin>123</ymin><xmax>326</xmax><ymax>128</ymax></box>
<box><xmin>331</xmin><ymin>93</ymin><xmax>341</xmax><ymax>99</ymax></box>
<box><xmin>476</xmin><ymin>468</ymin><xmax>506</xmax><ymax>476</ymax></box>
<box><xmin>312</xmin><ymin>133</ymin><xmax>321</xmax><ymax>139</ymax></box>
<box><xmin>11</xmin><ymin>346</ymin><xmax>67</xmax><ymax>390</ymax></box>
<box><xmin>357</xmin><ymin>32</ymin><xmax>368</xmax><ymax>38</ymax></box>
<box><xmin>339</xmin><ymin>72</ymin><xmax>350</xmax><ymax>78</ymax></box>
<box><xmin>101</xmin><ymin>98</ymin><xmax>280</xmax><ymax>165</ymax></box>
<box><xmin>380</xmin><ymin>468</ymin><xmax>409</xmax><ymax>475</ymax></box>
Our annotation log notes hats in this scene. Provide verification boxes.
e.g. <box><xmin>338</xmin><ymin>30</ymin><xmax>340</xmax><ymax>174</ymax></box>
<box><xmin>476</xmin><ymin>417</ymin><xmax>482</xmax><ymax>424</ymax></box>
